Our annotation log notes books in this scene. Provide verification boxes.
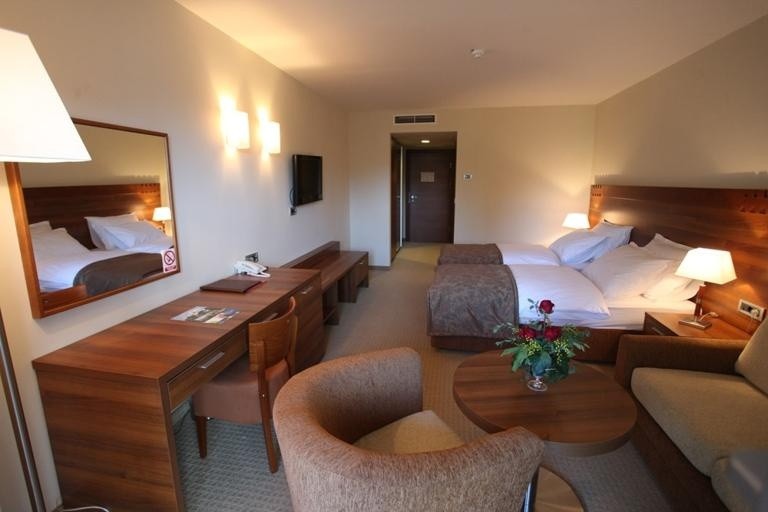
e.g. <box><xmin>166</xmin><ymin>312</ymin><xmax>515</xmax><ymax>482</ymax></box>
<box><xmin>200</xmin><ymin>279</ymin><xmax>262</xmax><ymax>294</ymax></box>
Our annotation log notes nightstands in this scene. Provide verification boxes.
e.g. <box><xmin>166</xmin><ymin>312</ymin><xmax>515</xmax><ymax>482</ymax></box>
<box><xmin>642</xmin><ymin>308</ymin><xmax>747</xmax><ymax>345</ymax></box>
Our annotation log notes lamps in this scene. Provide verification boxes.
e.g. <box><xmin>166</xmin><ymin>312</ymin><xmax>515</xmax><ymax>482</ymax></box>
<box><xmin>0</xmin><ymin>28</ymin><xmax>92</xmax><ymax>167</ymax></box>
<box><xmin>674</xmin><ymin>247</ymin><xmax>736</xmax><ymax>330</ymax></box>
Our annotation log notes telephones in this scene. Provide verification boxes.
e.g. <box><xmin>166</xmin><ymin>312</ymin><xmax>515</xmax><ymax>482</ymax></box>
<box><xmin>235</xmin><ymin>261</ymin><xmax>267</xmax><ymax>274</ymax></box>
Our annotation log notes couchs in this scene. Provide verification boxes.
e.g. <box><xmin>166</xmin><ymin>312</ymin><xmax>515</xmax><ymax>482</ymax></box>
<box><xmin>616</xmin><ymin>314</ymin><xmax>768</xmax><ymax>508</ymax></box>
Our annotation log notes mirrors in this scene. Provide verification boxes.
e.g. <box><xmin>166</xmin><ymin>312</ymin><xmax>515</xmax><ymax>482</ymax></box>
<box><xmin>5</xmin><ymin>116</ymin><xmax>180</xmax><ymax>323</ymax></box>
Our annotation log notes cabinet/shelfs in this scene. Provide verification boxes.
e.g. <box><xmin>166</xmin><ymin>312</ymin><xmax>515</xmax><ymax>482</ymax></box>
<box><xmin>283</xmin><ymin>240</ymin><xmax>370</xmax><ymax>327</ymax></box>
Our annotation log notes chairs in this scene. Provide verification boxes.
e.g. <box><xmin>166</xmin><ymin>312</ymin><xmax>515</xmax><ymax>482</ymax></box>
<box><xmin>190</xmin><ymin>294</ymin><xmax>301</xmax><ymax>477</ymax></box>
<box><xmin>272</xmin><ymin>345</ymin><xmax>549</xmax><ymax>510</ymax></box>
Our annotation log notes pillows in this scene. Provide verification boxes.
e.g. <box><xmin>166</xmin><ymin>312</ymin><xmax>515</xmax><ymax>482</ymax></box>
<box><xmin>549</xmin><ymin>212</ymin><xmax>632</xmax><ymax>265</ymax></box>
<box><xmin>580</xmin><ymin>234</ymin><xmax>706</xmax><ymax>305</ymax></box>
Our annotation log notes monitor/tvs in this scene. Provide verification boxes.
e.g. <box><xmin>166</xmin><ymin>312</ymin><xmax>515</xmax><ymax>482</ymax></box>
<box><xmin>293</xmin><ymin>154</ymin><xmax>323</xmax><ymax>207</ymax></box>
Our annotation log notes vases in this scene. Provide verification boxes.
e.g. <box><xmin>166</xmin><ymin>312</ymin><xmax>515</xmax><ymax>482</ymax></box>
<box><xmin>524</xmin><ymin>370</ymin><xmax>546</xmax><ymax>391</ymax></box>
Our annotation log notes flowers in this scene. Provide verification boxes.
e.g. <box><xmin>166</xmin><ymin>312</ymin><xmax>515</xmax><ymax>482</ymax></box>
<box><xmin>500</xmin><ymin>297</ymin><xmax>594</xmax><ymax>380</ymax></box>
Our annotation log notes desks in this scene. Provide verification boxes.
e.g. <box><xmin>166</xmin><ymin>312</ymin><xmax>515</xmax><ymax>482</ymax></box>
<box><xmin>30</xmin><ymin>266</ymin><xmax>328</xmax><ymax>512</ymax></box>
<box><xmin>454</xmin><ymin>354</ymin><xmax>638</xmax><ymax>512</ymax></box>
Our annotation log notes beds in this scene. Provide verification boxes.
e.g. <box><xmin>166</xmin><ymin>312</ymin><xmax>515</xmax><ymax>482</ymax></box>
<box><xmin>22</xmin><ymin>183</ymin><xmax>172</xmax><ymax>305</ymax></box>
<box><xmin>430</xmin><ymin>218</ymin><xmax>635</xmax><ymax>273</ymax></box>
<box><xmin>429</xmin><ymin>233</ymin><xmax>707</xmax><ymax>354</ymax></box>
